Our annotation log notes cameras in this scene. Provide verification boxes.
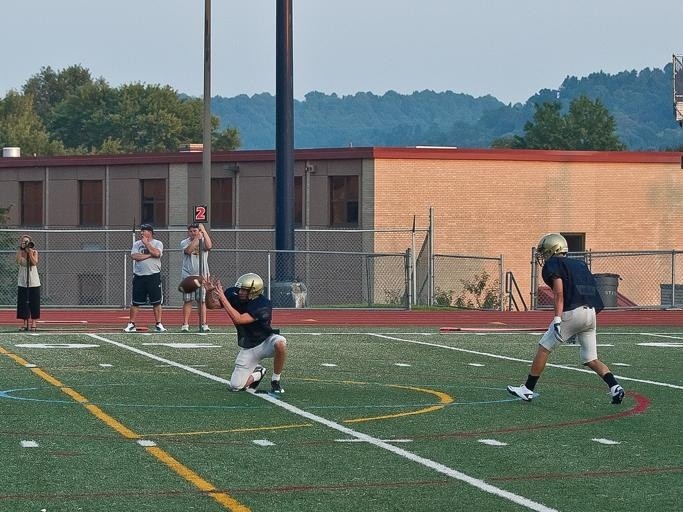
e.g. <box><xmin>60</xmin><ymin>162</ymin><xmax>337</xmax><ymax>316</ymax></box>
<box><xmin>24</xmin><ymin>240</ymin><xmax>35</xmax><ymax>249</ymax></box>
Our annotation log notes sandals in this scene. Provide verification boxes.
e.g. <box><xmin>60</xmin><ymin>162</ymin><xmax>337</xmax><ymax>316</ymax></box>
<box><xmin>19</xmin><ymin>327</ymin><xmax>37</xmax><ymax>331</ymax></box>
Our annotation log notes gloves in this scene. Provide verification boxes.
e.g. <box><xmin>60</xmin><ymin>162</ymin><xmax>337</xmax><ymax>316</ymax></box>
<box><xmin>553</xmin><ymin>316</ymin><xmax>564</xmax><ymax>343</ymax></box>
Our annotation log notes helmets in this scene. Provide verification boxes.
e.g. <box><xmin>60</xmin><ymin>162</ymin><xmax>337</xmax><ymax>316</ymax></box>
<box><xmin>235</xmin><ymin>273</ymin><xmax>264</xmax><ymax>300</ymax></box>
<box><xmin>535</xmin><ymin>233</ymin><xmax>569</xmax><ymax>267</ymax></box>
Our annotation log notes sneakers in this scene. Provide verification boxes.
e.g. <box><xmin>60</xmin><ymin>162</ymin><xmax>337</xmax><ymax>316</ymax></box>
<box><xmin>124</xmin><ymin>323</ymin><xmax>136</xmax><ymax>332</ymax></box>
<box><xmin>155</xmin><ymin>323</ymin><xmax>167</xmax><ymax>332</ymax></box>
<box><xmin>507</xmin><ymin>384</ymin><xmax>533</xmax><ymax>401</ymax></box>
<box><xmin>181</xmin><ymin>323</ymin><xmax>189</xmax><ymax>331</ymax></box>
<box><xmin>200</xmin><ymin>324</ymin><xmax>211</xmax><ymax>331</ymax></box>
<box><xmin>271</xmin><ymin>379</ymin><xmax>285</xmax><ymax>394</ymax></box>
<box><xmin>248</xmin><ymin>367</ymin><xmax>266</xmax><ymax>389</ymax></box>
<box><xmin>610</xmin><ymin>385</ymin><xmax>625</xmax><ymax>404</ymax></box>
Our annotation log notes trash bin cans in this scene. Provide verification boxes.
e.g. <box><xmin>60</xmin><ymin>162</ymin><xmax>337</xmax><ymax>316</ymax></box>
<box><xmin>593</xmin><ymin>273</ymin><xmax>619</xmax><ymax>306</ymax></box>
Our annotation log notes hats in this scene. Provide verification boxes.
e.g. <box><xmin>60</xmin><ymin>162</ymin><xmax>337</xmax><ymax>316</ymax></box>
<box><xmin>141</xmin><ymin>224</ymin><xmax>154</xmax><ymax>231</ymax></box>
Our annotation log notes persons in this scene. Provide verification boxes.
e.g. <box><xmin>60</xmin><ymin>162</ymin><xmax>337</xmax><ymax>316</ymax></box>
<box><xmin>196</xmin><ymin>272</ymin><xmax>288</xmax><ymax>394</ymax></box>
<box><xmin>177</xmin><ymin>223</ymin><xmax>214</xmax><ymax>331</ymax></box>
<box><xmin>506</xmin><ymin>232</ymin><xmax>626</xmax><ymax>405</ymax></box>
<box><xmin>123</xmin><ymin>224</ymin><xmax>167</xmax><ymax>332</ymax></box>
<box><xmin>12</xmin><ymin>234</ymin><xmax>40</xmax><ymax>333</ymax></box>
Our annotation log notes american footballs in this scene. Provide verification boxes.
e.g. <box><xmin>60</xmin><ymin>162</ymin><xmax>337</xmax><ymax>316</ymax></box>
<box><xmin>178</xmin><ymin>276</ymin><xmax>202</xmax><ymax>292</ymax></box>
